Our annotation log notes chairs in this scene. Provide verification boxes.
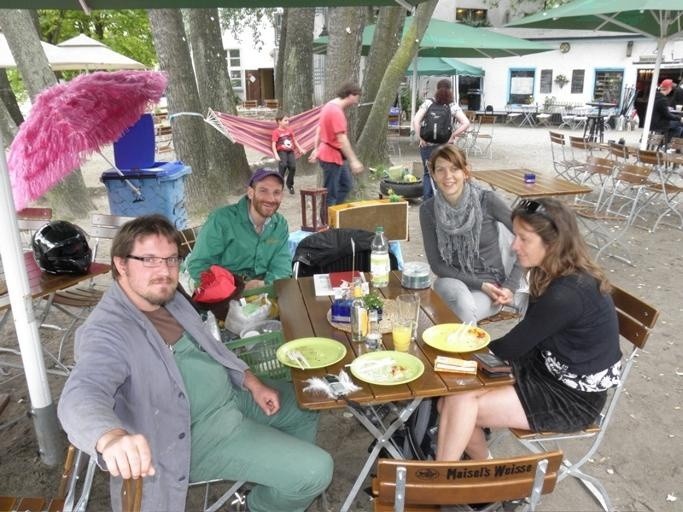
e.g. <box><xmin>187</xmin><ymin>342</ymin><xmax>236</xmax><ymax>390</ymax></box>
<box><xmin>373</xmin><ymin>451</ymin><xmax>563</xmax><ymax>512</ymax></box>
<box><xmin>454</xmin><ymin>104</ymin><xmax>682</xmax><ymax>268</ymax></box>
<box><xmin>488</xmin><ymin>281</ymin><xmax>660</xmax><ymax>512</ymax></box>
<box><xmin>244</xmin><ymin>99</ymin><xmax>279</xmax><ymax>115</ymax></box>
<box><xmin>386</xmin><ymin>108</ymin><xmax>403</xmax><ymax>136</ymax></box>
<box><xmin>153</xmin><ymin>111</ymin><xmax>173</xmax><ymax>162</ymax></box>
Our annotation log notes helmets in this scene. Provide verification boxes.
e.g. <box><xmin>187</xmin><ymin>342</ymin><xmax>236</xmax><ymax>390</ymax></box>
<box><xmin>30</xmin><ymin>219</ymin><xmax>93</xmax><ymax>277</ymax></box>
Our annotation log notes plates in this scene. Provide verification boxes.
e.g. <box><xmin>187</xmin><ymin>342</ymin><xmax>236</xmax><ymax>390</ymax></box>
<box><xmin>422</xmin><ymin>323</ymin><xmax>490</xmax><ymax>353</ymax></box>
<box><xmin>350</xmin><ymin>349</ymin><xmax>425</xmax><ymax>385</ymax></box>
<box><xmin>276</xmin><ymin>337</ymin><xmax>347</xmax><ymax>369</ymax></box>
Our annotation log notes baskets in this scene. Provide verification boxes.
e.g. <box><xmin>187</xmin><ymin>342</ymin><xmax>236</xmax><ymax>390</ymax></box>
<box><xmin>203</xmin><ymin>285</ymin><xmax>296</xmax><ymax>385</ymax></box>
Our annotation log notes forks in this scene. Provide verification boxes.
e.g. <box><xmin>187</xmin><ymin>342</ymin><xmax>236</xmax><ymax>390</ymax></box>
<box><xmin>286</xmin><ymin>350</ymin><xmax>304</xmax><ymax>369</ymax></box>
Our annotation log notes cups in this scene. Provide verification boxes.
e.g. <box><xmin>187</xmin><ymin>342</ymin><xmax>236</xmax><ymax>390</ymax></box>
<box><xmin>239</xmin><ymin>319</ymin><xmax>283</xmax><ymax>362</ymax></box>
<box><xmin>389</xmin><ymin>292</ymin><xmax>420</xmax><ymax>351</ymax></box>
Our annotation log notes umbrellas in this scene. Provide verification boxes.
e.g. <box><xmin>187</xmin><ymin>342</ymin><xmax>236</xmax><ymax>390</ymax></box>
<box><xmin>5</xmin><ymin>70</ymin><xmax>169</xmax><ymax>212</ymax></box>
<box><xmin>39</xmin><ymin>31</ymin><xmax>148</xmax><ymax>69</ymax></box>
<box><xmin>304</xmin><ymin>13</ymin><xmax>558</xmax><ymax>134</ymax></box>
<box><xmin>502</xmin><ymin>2</ymin><xmax>683</xmax><ymax>150</ymax></box>
<box><xmin>373</xmin><ymin>55</ymin><xmax>485</xmax><ymax>79</ymax></box>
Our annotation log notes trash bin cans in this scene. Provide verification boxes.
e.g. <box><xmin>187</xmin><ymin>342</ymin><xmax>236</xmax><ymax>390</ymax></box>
<box><xmin>102</xmin><ymin>161</ymin><xmax>192</xmax><ymax>231</ymax></box>
<box><xmin>466</xmin><ymin>89</ymin><xmax>481</xmax><ymax>111</ymax></box>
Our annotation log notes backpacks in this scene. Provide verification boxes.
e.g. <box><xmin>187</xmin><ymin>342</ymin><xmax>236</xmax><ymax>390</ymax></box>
<box><xmin>420</xmin><ymin>96</ymin><xmax>457</xmax><ymax>145</ymax></box>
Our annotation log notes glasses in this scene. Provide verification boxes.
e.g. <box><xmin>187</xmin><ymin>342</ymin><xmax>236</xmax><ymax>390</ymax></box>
<box><xmin>124</xmin><ymin>254</ymin><xmax>184</xmax><ymax>267</ymax></box>
<box><xmin>515</xmin><ymin>197</ymin><xmax>559</xmax><ymax>227</ymax></box>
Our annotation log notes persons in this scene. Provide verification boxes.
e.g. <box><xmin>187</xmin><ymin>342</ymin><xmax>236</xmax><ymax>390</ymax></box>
<box><xmin>648</xmin><ymin>77</ymin><xmax>683</xmax><ymax>155</ymax></box>
<box><xmin>427</xmin><ymin>191</ymin><xmax>624</xmax><ymax>462</ymax></box>
<box><xmin>53</xmin><ymin>212</ymin><xmax>336</xmax><ymax>510</ymax></box>
<box><xmin>417</xmin><ymin>143</ymin><xmax>530</xmax><ymax>326</ymax></box>
<box><xmin>181</xmin><ymin>166</ymin><xmax>297</xmax><ymax>294</ymax></box>
<box><xmin>307</xmin><ymin>83</ymin><xmax>367</xmax><ymax>208</ymax></box>
<box><xmin>411</xmin><ymin>79</ymin><xmax>471</xmax><ymax>201</ymax></box>
<box><xmin>270</xmin><ymin>112</ymin><xmax>307</xmax><ymax>193</ymax></box>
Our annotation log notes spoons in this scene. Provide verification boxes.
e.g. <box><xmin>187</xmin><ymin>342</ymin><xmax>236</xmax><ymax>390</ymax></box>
<box><xmin>294</xmin><ymin>350</ymin><xmax>311</xmax><ymax>368</ymax></box>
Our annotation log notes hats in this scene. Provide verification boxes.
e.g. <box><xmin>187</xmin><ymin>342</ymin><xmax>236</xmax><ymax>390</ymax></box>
<box><xmin>661</xmin><ymin>78</ymin><xmax>678</xmax><ymax>89</ymax></box>
<box><xmin>248</xmin><ymin>167</ymin><xmax>285</xmax><ymax>190</ymax></box>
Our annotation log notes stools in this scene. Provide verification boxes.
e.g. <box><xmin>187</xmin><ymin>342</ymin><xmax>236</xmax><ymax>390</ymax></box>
<box><xmin>300</xmin><ymin>186</ymin><xmax>329</xmax><ymax>231</ymax></box>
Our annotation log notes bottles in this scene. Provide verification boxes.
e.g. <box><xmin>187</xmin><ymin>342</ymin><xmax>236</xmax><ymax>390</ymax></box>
<box><xmin>371</xmin><ymin>226</ymin><xmax>391</xmax><ymax>287</ymax></box>
<box><xmin>349</xmin><ymin>275</ymin><xmax>384</xmax><ymax>351</ymax></box>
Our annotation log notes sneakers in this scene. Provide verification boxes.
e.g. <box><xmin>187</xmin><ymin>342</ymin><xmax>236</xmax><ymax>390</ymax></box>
<box><xmin>286</xmin><ymin>182</ymin><xmax>295</xmax><ymax>195</ymax></box>
